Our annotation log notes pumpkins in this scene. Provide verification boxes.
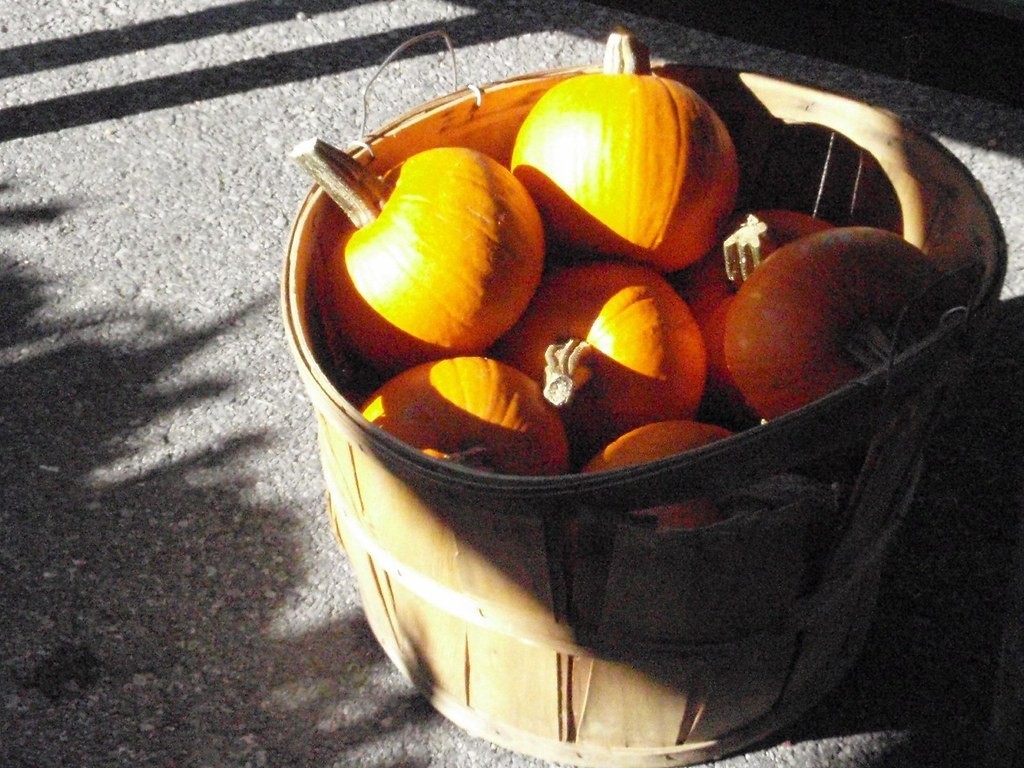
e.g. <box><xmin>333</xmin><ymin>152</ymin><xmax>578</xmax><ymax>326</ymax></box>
<box><xmin>289</xmin><ymin>23</ymin><xmax>956</xmax><ymax>478</ymax></box>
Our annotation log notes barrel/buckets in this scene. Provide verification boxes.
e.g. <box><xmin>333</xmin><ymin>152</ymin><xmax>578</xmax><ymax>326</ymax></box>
<box><xmin>279</xmin><ymin>28</ymin><xmax>1009</xmax><ymax>768</ymax></box>
